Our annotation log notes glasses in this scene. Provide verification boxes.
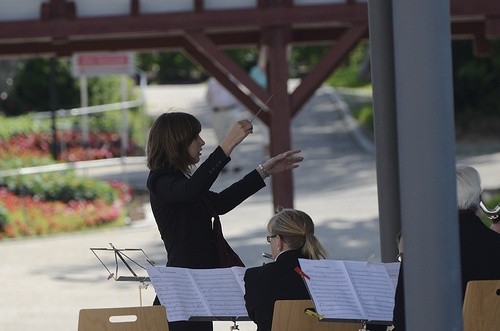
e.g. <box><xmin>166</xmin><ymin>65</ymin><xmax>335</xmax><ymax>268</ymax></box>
<box><xmin>267</xmin><ymin>234</ymin><xmax>277</xmax><ymax>242</ymax></box>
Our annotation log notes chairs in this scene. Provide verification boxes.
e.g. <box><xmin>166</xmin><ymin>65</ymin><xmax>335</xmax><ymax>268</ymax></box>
<box><xmin>462</xmin><ymin>280</ymin><xmax>500</xmax><ymax>331</ymax></box>
<box><xmin>78</xmin><ymin>306</ymin><xmax>170</xmax><ymax>331</ymax></box>
<box><xmin>271</xmin><ymin>299</ymin><xmax>362</xmax><ymax>330</ymax></box>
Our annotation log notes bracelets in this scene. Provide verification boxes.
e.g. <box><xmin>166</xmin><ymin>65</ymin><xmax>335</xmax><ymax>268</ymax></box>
<box><xmin>259</xmin><ymin>164</ymin><xmax>270</xmax><ymax>177</ymax></box>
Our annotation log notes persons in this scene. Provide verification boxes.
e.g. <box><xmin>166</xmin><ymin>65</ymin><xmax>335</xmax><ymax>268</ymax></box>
<box><xmin>243</xmin><ymin>208</ymin><xmax>327</xmax><ymax>331</ymax></box>
<box><xmin>203</xmin><ymin>74</ymin><xmax>243</xmax><ymax>174</ymax></box>
<box><xmin>144</xmin><ymin>112</ymin><xmax>306</xmax><ymax>331</ymax></box>
<box><xmin>248</xmin><ymin>54</ymin><xmax>267</xmax><ymax>90</ymax></box>
<box><xmin>392</xmin><ymin>166</ymin><xmax>500</xmax><ymax>331</ymax></box>
